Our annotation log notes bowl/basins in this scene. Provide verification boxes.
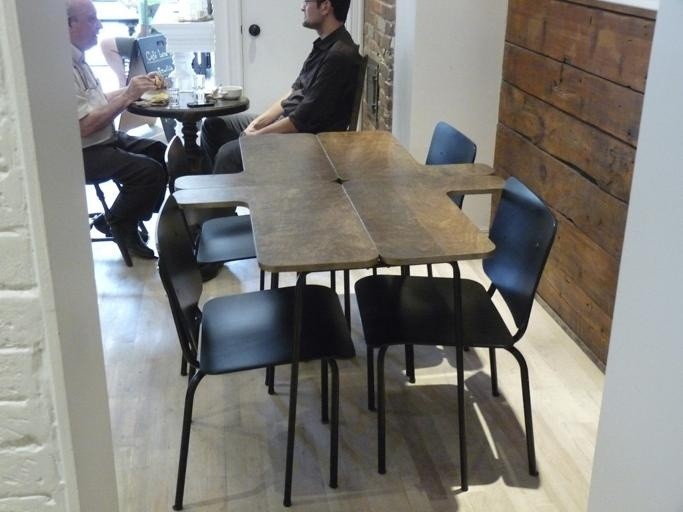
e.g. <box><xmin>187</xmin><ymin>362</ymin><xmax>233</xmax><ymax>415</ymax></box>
<box><xmin>220</xmin><ymin>85</ymin><xmax>242</xmax><ymax>100</ymax></box>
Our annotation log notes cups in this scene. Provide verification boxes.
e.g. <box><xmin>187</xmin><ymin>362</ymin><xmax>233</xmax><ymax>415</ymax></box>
<box><xmin>168</xmin><ymin>89</ymin><xmax>179</xmax><ymax>106</ymax></box>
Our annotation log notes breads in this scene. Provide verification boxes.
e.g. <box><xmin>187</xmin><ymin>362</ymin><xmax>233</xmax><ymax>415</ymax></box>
<box><xmin>151</xmin><ymin>76</ymin><xmax>162</xmax><ymax>89</ymax></box>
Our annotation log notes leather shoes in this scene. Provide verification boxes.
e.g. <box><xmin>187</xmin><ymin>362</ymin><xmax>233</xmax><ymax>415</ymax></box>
<box><xmin>94</xmin><ymin>213</ymin><xmax>155</xmax><ymax>259</ymax></box>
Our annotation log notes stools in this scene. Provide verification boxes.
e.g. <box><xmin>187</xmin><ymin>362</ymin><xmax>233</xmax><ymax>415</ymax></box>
<box><xmin>85</xmin><ymin>177</ymin><xmax>149</xmax><ymax>267</ymax></box>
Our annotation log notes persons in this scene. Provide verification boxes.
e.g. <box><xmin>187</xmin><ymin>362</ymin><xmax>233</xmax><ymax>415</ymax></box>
<box><xmin>65</xmin><ymin>0</ymin><xmax>167</xmax><ymax>259</ymax></box>
<box><xmin>200</xmin><ymin>0</ymin><xmax>363</xmax><ymax>282</ymax></box>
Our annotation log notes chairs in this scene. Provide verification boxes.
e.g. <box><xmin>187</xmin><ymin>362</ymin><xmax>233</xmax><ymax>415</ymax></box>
<box><xmin>366</xmin><ymin>121</ymin><xmax>478</xmax><ymax>354</ymax></box>
<box><xmin>153</xmin><ymin>194</ymin><xmax>358</xmax><ymax>511</ymax></box>
<box><xmin>162</xmin><ymin>133</ymin><xmax>285</xmax><ymax>289</ymax></box>
<box><xmin>354</xmin><ymin>173</ymin><xmax>556</xmax><ymax>493</ymax></box>
<box><xmin>348</xmin><ymin>54</ymin><xmax>369</xmax><ymax>131</ymax></box>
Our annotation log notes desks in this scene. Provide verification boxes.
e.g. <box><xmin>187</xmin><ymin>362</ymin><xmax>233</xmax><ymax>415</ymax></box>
<box><xmin>172</xmin><ymin>130</ymin><xmax>508</xmax><ymax>395</ymax></box>
<box><xmin>127</xmin><ymin>88</ymin><xmax>250</xmax><ymax>163</ymax></box>
